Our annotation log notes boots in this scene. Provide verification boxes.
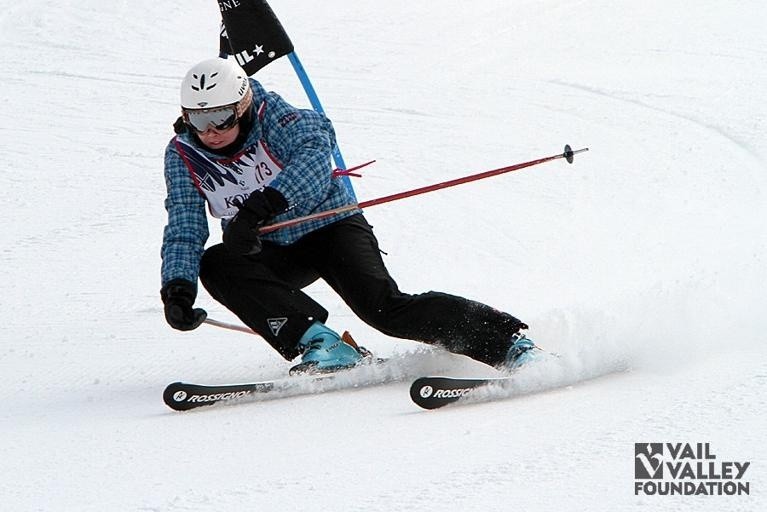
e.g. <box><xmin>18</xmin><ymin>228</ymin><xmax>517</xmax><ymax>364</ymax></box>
<box><xmin>503</xmin><ymin>332</ymin><xmax>563</xmax><ymax>375</ymax></box>
<box><xmin>289</xmin><ymin>321</ymin><xmax>363</xmax><ymax>377</ymax></box>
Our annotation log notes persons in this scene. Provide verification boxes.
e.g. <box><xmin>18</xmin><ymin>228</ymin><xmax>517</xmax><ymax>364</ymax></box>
<box><xmin>160</xmin><ymin>57</ymin><xmax>565</xmax><ymax>378</ymax></box>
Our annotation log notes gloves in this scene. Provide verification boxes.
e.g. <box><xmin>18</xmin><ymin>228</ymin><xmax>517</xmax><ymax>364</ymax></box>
<box><xmin>223</xmin><ymin>185</ymin><xmax>288</xmax><ymax>256</ymax></box>
<box><xmin>160</xmin><ymin>278</ymin><xmax>207</xmax><ymax>331</ymax></box>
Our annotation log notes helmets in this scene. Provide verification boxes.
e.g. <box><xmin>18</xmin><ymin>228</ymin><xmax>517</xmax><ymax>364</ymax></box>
<box><xmin>181</xmin><ymin>57</ymin><xmax>253</xmax><ymax>134</ymax></box>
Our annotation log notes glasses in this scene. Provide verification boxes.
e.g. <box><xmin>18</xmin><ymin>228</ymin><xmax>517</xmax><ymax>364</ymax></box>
<box><xmin>182</xmin><ymin>87</ymin><xmax>253</xmax><ymax>137</ymax></box>
